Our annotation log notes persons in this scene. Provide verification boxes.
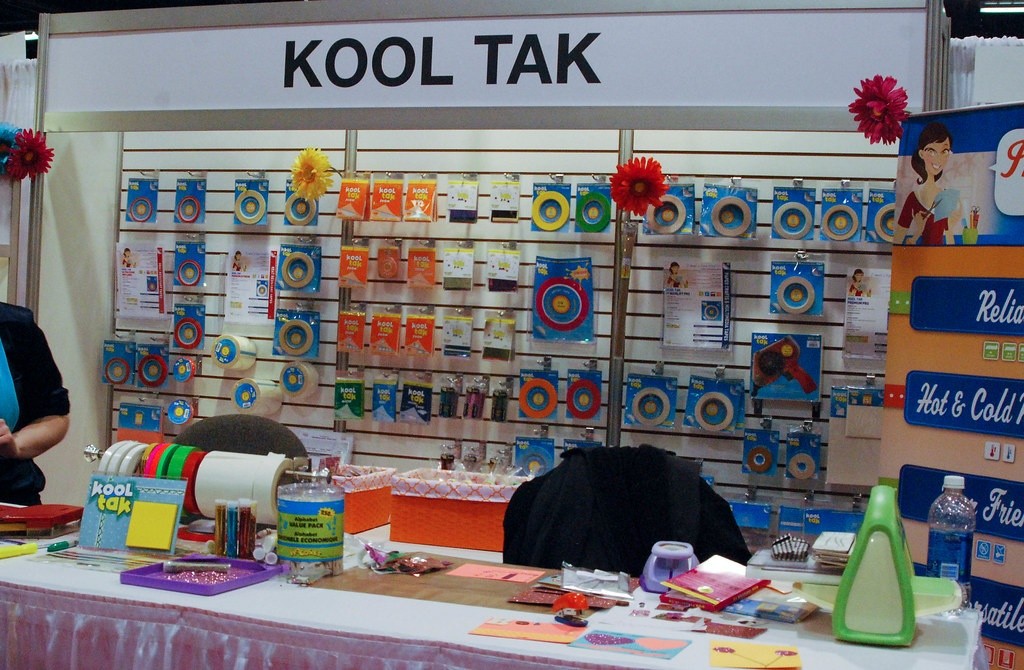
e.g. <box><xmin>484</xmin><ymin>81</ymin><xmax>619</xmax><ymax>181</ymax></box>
<box><xmin>0</xmin><ymin>301</ymin><xmax>71</xmax><ymax>508</ymax></box>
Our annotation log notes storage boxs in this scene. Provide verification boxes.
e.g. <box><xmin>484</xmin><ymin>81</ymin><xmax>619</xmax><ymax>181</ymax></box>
<box><xmin>337</xmin><ymin>246</ymin><xmax>370</xmax><ymax>289</ymax></box>
<box><xmin>389</xmin><ymin>467</ymin><xmax>529</xmax><ymax>551</ymax></box>
<box><xmin>335</xmin><ymin>178</ymin><xmax>371</xmax><ymax>222</ymax></box>
<box><xmin>369</xmin><ymin>312</ymin><xmax>402</xmax><ymax>357</ymax></box>
<box><xmin>486</xmin><ymin>248</ymin><xmax>521</xmax><ymax>294</ymax></box>
<box><xmin>337</xmin><ymin>310</ymin><xmax>366</xmax><ymax>354</ymax></box>
<box><xmin>489</xmin><ymin>180</ymin><xmax>521</xmax><ymax>224</ymax></box>
<box><xmin>405</xmin><ymin>314</ymin><xmax>436</xmax><ymax>358</ymax></box>
<box><xmin>445</xmin><ymin>180</ymin><xmax>479</xmax><ymax>224</ymax></box>
<box><xmin>442</xmin><ymin>247</ymin><xmax>475</xmax><ymax>294</ymax></box>
<box><xmin>377</xmin><ymin>245</ymin><xmax>401</xmax><ymax>278</ymax></box>
<box><xmin>482</xmin><ymin>318</ymin><xmax>517</xmax><ymax>362</ymax></box>
<box><xmin>369</xmin><ymin>179</ymin><xmax>402</xmax><ymax>222</ymax></box>
<box><xmin>403</xmin><ymin>179</ymin><xmax>439</xmax><ymax>223</ymax></box>
<box><xmin>312</xmin><ymin>465</ymin><xmax>398</xmax><ymax>535</ymax></box>
<box><xmin>407</xmin><ymin>247</ymin><xmax>437</xmax><ymax>289</ymax></box>
<box><xmin>442</xmin><ymin>316</ymin><xmax>474</xmax><ymax>358</ymax></box>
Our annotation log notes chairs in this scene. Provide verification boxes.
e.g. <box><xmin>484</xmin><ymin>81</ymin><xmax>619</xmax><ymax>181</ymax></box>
<box><xmin>503</xmin><ymin>444</ymin><xmax>752</xmax><ymax>578</ymax></box>
<box><xmin>171</xmin><ymin>413</ymin><xmax>312</xmax><ymax>533</ymax></box>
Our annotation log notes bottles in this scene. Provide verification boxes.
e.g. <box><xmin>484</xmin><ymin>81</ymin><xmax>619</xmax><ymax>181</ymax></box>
<box><xmin>925</xmin><ymin>475</ymin><xmax>975</xmax><ymax>619</ymax></box>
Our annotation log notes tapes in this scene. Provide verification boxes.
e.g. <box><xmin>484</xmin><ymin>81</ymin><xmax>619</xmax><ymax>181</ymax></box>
<box><xmin>235</xmin><ymin>190</ymin><xmax>266</xmax><ymax>225</ymax></box>
<box><xmin>130</xmin><ymin>198</ymin><xmax>152</xmax><ymax>222</ymax></box>
<box><xmin>646</xmin><ymin>194</ymin><xmax>896</xmax><ymax>245</ymax></box>
<box><xmin>518</xmin><ymin>445</ymin><xmax>553</xmax><ymax>479</ymax></box>
<box><xmin>211</xmin><ymin>334</ymin><xmax>257</xmax><ymax>371</ymax></box>
<box><xmin>279</xmin><ymin>361</ymin><xmax>319</xmax><ymax>399</ymax></box>
<box><xmin>178</xmin><ymin>196</ymin><xmax>200</xmax><ymax>223</ymax></box>
<box><xmin>178</xmin><ymin>260</ymin><xmax>202</xmax><ymax>286</ymax></box>
<box><xmin>631</xmin><ymin>386</ymin><xmax>736</xmax><ymax>430</ymax></box>
<box><xmin>279</xmin><ymin>319</ymin><xmax>314</xmax><ymax>356</ymax></box>
<box><xmin>138</xmin><ymin>354</ymin><xmax>167</xmax><ymax>387</ymax></box>
<box><xmin>106</xmin><ymin>358</ymin><xmax>130</xmax><ymax>385</ymax></box>
<box><xmin>173</xmin><ymin>356</ymin><xmax>195</xmax><ymax>383</ymax></box>
<box><xmin>776</xmin><ymin>276</ymin><xmax>815</xmax><ymax>315</ymax></box>
<box><xmin>175</xmin><ymin>318</ymin><xmax>202</xmax><ymax>348</ymax></box>
<box><xmin>285</xmin><ymin>192</ymin><xmax>317</xmax><ymax>226</ymax></box>
<box><xmin>282</xmin><ymin>252</ymin><xmax>315</xmax><ymax>289</ymax></box>
<box><xmin>168</xmin><ymin>399</ymin><xmax>195</xmax><ymax>425</ymax></box>
<box><xmin>749</xmin><ymin>447</ymin><xmax>815</xmax><ymax>481</ymax></box>
<box><xmin>516</xmin><ymin>189</ymin><xmax>613</xmax><ymax>423</ymax></box>
<box><xmin>231</xmin><ymin>378</ymin><xmax>282</xmax><ymax>414</ymax></box>
<box><xmin>97</xmin><ymin>439</ymin><xmax>293</xmax><ymax>526</ymax></box>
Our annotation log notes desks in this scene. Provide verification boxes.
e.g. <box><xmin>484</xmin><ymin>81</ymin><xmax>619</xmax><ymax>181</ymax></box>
<box><xmin>0</xmin><ymin>503</ymin><xmax>989</xmax><ymax>670</ymax></box>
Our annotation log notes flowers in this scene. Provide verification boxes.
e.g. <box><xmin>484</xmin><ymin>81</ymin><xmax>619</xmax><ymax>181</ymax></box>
<box><xmin>0</xmin><ymin>121</ymin><xmax>23</xmax><ymax>175</ymax></box>
<box><xmin>2</xmin><ymin>127</ymin><xmax>54</xmax><ymax>182</ymax></box>
<box><xmin>289</xmin><ymin>147</ymin><xmax>344</xmax><ymax>202</ymax></box>
<box><xmin>848</xmin><ymin>74</ymin><xmax>914</xmax><ymax>147</ymax></box>
<box><xmin>609</xmin><ymin>155</ymin><xmax>670</xmax><ymax>216</ymax></box>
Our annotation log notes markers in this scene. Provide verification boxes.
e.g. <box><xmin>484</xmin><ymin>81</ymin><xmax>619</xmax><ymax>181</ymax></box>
<box><xmin>47</xmin><ymin>540</ymin><xmax>79</xmax><ymax>552</ymax></box>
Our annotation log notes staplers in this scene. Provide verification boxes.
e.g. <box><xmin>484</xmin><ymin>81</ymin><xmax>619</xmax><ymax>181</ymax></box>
<box><xmin>551</xmin><ymin>592</ymin><xmax>589</xmax><ymax>626</ymax></box>
